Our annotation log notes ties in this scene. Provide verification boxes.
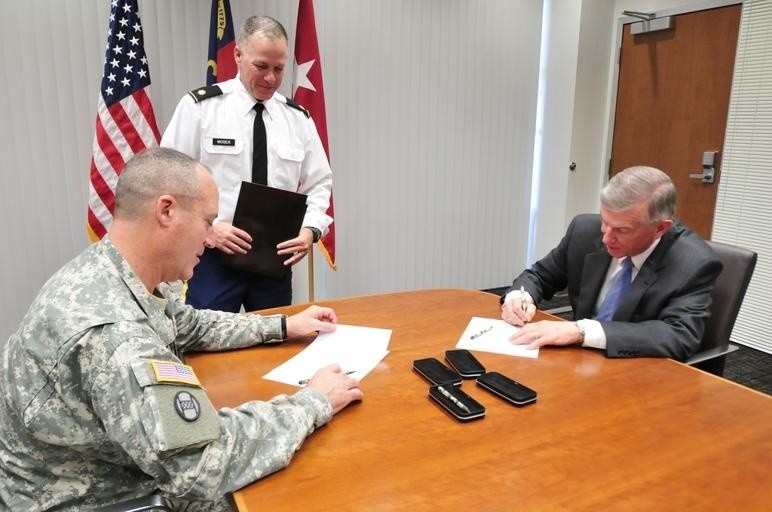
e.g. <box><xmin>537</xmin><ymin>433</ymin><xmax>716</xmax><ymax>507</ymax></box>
<box><xmin>591</xmin><ymin>256</ymin><xmax>634</xmax><ymax>321</ymax></box>
<box><xmin>252</xmin><ymin>103</ymin><xmax>267</xmax><ymax>186</ymax></box>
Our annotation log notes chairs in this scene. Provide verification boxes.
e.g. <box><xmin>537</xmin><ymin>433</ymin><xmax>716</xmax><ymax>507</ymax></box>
<box><xmin>542</xmin><ymin>240</ymin><xmax>758</xmax><ymax>376</ymax></box>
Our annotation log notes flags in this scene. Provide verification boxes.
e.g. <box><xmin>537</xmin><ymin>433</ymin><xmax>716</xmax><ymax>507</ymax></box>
<box><xmin>85</xmin><ymin>0</ymin><xmax>162</xmax><ymax>243</ymax></box>
<box><xmin>205</xmin><ymin>0</ymin><xmax>239</xmax><ymax>87</ymax></box>
<box><xmin>289</xmin><ymin>0</ymin><xmax>337</xmax><ymax>269</ymax></box>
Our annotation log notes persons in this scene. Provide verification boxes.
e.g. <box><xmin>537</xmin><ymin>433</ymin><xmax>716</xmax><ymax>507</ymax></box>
<box><xmin>498</xmin><ymin>165</ymin><xmax>723</xmax><ymax>364</ymax></box>
<box><xmin>1</xmin><ymin>145</ymin><xmax>366</xmax><ymax>511</ymax></box>
<box><xmin>158</xmin><ymin>13</ymin><xmax>334</xmax><ymax>314</ymax></box>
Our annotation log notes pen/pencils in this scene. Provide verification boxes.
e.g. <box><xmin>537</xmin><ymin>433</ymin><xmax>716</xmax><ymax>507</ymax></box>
<box><xmin>521</xmin><ymin>285</ymin><xmax>527</xmax><ymax>323</ymax></box>
<box><xmin>298</xmin><ymin>370</ymin><xmax>357</xmax><ymax>385</ymax></box>
<box><xmin>469</xmin><ymin>324</ymin><xmax>493</xmax><ymax>339</ymax></box>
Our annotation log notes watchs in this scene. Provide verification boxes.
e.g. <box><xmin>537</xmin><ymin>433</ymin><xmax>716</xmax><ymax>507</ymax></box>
<box><xmin>574</xmin><ymin>317</ymin><xmax>584</xmax><ymax>346</ymax></box>
<box><xmin>304</xmin><ymin>225</ymin><xmax>321</xmax><ymax>244</ymax></box>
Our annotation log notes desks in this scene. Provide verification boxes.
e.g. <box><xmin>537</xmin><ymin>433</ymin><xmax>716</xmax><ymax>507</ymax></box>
<box><xmin>184</xmin><ymin>287</ymin><xmax>772</xmax><ymax>512</ymax></box>
